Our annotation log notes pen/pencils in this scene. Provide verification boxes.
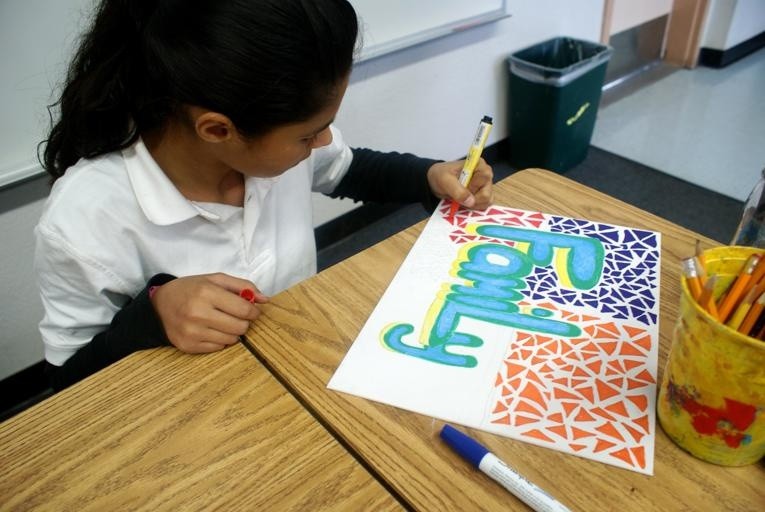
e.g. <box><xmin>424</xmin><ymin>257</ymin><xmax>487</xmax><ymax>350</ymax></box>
<box><xmin>439</xmin><ymin>424</ymin><xmax>572</xmax><ymax>512</ymax></box>
<box><xmin>450</xmin><ymin>116</ymin><xmax>493</xmax><ymax>217</ymax></box>
<box><xmin>682</xmin><ymin>239</ymin><xmax>765</xmax><ymax>341</ymax></box>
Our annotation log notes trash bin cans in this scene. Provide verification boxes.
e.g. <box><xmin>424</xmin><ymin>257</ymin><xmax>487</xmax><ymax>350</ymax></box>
<box><xmin>508</xmin><ymin>37</ymin><xmax>609</xmax><ymax>174</ymax></box>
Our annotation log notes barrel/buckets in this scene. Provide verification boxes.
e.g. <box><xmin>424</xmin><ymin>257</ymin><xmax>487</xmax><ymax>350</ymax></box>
<box><xmin>656</xmin><ymin>244</ymin><xmax>765</xmax><ymax>467</ymax></box>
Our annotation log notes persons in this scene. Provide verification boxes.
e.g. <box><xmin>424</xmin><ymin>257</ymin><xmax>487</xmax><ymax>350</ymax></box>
<box><xmin>30</xmin><ymin>0</ymin><xmax>495</xmax><ymax>395</ymax></box>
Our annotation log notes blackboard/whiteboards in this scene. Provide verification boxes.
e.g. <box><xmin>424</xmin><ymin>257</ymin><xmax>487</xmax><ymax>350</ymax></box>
<box><xmin>0</xmin><ymin>0</ymin><xmax>510</xmax><ymax>188</ymax></box>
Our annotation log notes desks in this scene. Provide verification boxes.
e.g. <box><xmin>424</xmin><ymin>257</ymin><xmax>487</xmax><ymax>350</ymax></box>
<box><xmin>0</xmin><ymin>341</ymin><xmax>412</xmax><ymax>512</ymax></box>
<box><xmin>244</xmin><ymin>168</ymin><xmax>765</xmax><ymax>512</ymax></box>
